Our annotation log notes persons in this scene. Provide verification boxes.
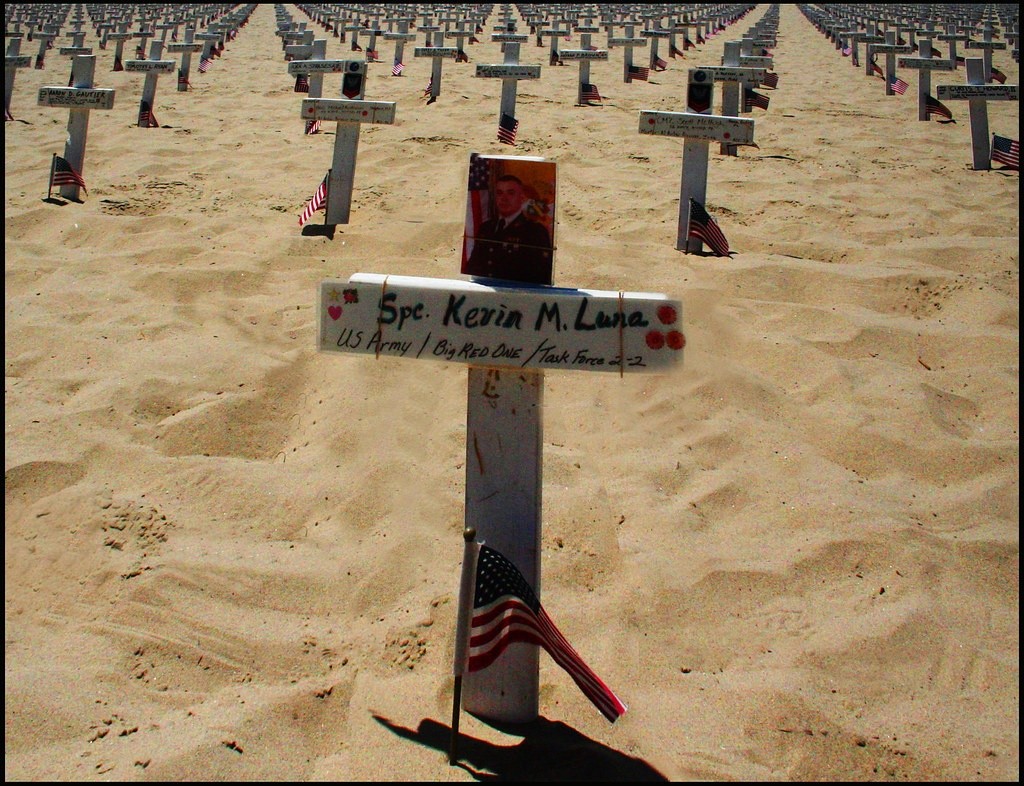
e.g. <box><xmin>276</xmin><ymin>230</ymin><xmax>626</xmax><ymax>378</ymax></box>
<box><xmin>464</xmin><ymin>173</ymin><xmax>551</xmax><ymax>281</ymax></box>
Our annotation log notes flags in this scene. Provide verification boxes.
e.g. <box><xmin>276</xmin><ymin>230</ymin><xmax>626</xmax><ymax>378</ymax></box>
<box><xmin>454</xmin><ymin>541</ymin><xmax>627</xmax><ymax>725</ymax></box>
<box><xmin>5</xmin><ymin>1</ymin><xmax>1019</xmax><ymax>253</ymax></box>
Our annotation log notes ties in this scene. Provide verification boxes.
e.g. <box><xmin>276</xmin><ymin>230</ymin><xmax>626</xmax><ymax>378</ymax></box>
<box><xmin>496</xmin><ymin>218</ymin><xmax>507</xmax><ymax>237</ymax></box>
